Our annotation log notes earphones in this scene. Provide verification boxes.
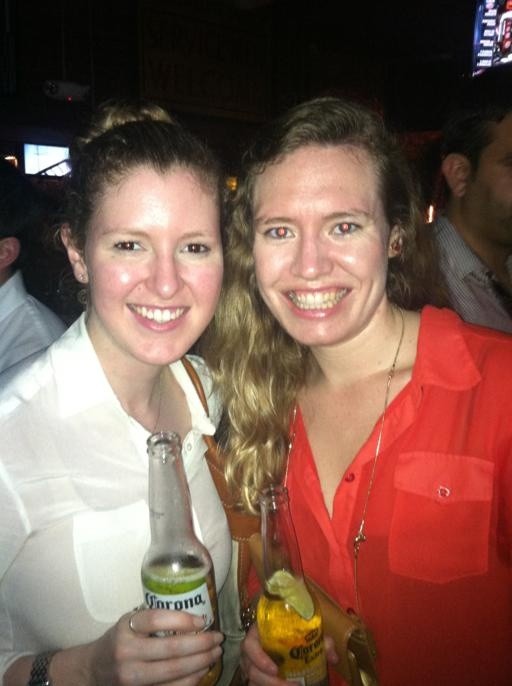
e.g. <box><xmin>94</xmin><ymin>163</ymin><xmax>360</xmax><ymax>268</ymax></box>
<box><xmin>390</xmin><ymin>228</ymin><xmax>402</xmax><ymax>252</ymax></box>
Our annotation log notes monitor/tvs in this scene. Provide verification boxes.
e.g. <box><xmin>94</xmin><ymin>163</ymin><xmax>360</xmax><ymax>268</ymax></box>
<box><xmin>23</xmin><ymin>145</ymin><xmax>72</xmax><ymax>182</ymax></box>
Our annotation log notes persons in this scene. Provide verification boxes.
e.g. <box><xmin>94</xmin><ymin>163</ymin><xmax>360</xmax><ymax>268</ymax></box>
<box><xmin>0</xmin><ymin>95</ymin><xmax>512</xmax><ymax>686</ymax></box>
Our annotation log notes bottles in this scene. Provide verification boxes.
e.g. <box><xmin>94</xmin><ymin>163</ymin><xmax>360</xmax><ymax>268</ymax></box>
<box><xmin>253</xmin><ymin>480</ymin><xmax>331</xmax><ymax>681</ymax></box>
<box><xmin>139</xmin><ymin>431</ymin><xmax>221</xmax><ymax>685</ymax></box>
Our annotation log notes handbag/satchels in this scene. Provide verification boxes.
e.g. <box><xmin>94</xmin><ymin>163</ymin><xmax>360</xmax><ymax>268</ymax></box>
<box><xmin>250</xmin><ymin>533</ymin><xmax>378</xmax><ymax>685</ymax></box>
<box><xmin>203</xmin><ymin>442</ymin><xmax>273</xmax><ymax>686</ymax></box>
